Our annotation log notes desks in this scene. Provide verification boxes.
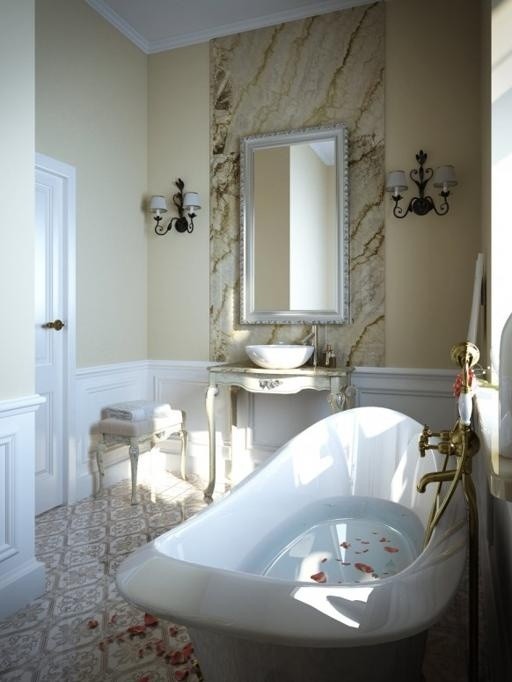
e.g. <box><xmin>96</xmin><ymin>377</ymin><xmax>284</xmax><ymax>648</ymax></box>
<box><xmin>204</xmin><ymin>361</ymin><xmax>357</xmax><ymax>505</ymax></box>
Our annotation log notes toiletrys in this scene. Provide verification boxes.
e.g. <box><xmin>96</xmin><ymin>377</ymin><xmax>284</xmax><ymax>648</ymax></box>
<box><xmin>325</xmin><ymin>343</ymin><xmax>335</xmax><ymax>368</ymax></box>
<box><xmin>317</xmin><ymin>345</ymin><xmax>336</xmax><ymax>368</ymax></box>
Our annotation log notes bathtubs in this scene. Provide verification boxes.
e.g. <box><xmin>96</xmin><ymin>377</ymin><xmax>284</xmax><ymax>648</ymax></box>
<box><xmin>116</xmin><ymin>404</ymin><xmax>470</xmax><ymax>682</ymax></box>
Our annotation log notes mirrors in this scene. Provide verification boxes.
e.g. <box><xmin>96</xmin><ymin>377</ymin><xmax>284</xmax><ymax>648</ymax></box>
<box><xmin>240</xmin><ymin>123</ymin><xmax>350</xmax><ymax>326</ymax></box>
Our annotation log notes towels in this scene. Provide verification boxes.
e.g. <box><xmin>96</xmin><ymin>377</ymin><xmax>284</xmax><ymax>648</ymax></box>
<box><xmin>101</xmin><ymin>398</ymin><xmax>171</xmax><ymax>422</ymax></box>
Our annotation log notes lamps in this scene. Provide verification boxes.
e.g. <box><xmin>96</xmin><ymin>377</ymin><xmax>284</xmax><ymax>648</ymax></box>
<box><xmin>382</xmin><ymin>149</ymin><xmax>457</xmax><ymax>220</ymax></box>
<box><xmin>147</xmin><ymin>177</ymin><xmax>203</xmax><ymax>236</ymax></box>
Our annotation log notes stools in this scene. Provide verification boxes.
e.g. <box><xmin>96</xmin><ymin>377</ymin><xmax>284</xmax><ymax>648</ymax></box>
<box><xmin>90</xmin><ymin>410</ymin><xmax>189</xmax><ymax>507</ymax></box>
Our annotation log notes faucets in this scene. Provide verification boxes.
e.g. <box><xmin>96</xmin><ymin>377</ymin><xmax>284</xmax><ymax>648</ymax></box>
<box><xmin>415</xmin><ymin>421</ymin><xmax>456</xmax><ymax>457</ymax></box>
<box><xmin>300</xmin><ymin>322</ymin><xmax>318</xmax><ymax>366</ymax></box>
<box><xmin>417</xmin><ymin>470</ymin><xmax>459</xmax><ymax>492</ymax></box>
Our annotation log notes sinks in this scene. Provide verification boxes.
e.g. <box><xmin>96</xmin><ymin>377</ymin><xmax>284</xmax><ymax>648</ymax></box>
<box><xmin>245</xmin><ymin>343</ymin><xmax>316</xmax><ymax>369</ymax></box>
